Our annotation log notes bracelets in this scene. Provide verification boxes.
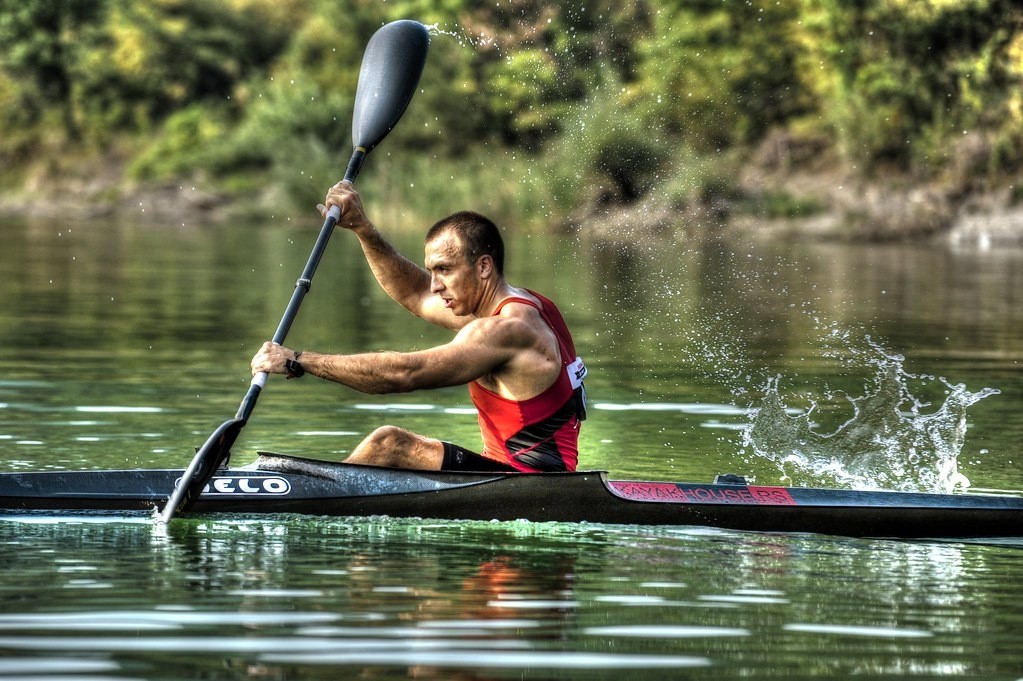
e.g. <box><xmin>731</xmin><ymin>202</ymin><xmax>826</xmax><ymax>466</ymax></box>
<box><xmin>285</xmin><ymin>351</ymin><xmax>306</xmax><ymax>379</ymax></box>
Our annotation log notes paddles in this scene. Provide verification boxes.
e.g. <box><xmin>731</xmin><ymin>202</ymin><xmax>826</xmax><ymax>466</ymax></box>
<box><xmin>161</xmin><ymin>19</ymin><xmax>429</xmax><ymax>518</ymax></box>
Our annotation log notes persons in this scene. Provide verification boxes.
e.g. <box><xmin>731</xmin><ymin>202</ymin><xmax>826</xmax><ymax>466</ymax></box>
<box><xmin>250</xmin><ymin>179</ymin><xmax>588</xmax><ymax>472</ymax></box>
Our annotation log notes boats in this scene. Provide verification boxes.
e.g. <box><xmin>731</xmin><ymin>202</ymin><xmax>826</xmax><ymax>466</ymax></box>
<box><xmin>0</xmin><ymin>455</ymin><xmax>1023</xmax><ymax>538</ymax></box>
